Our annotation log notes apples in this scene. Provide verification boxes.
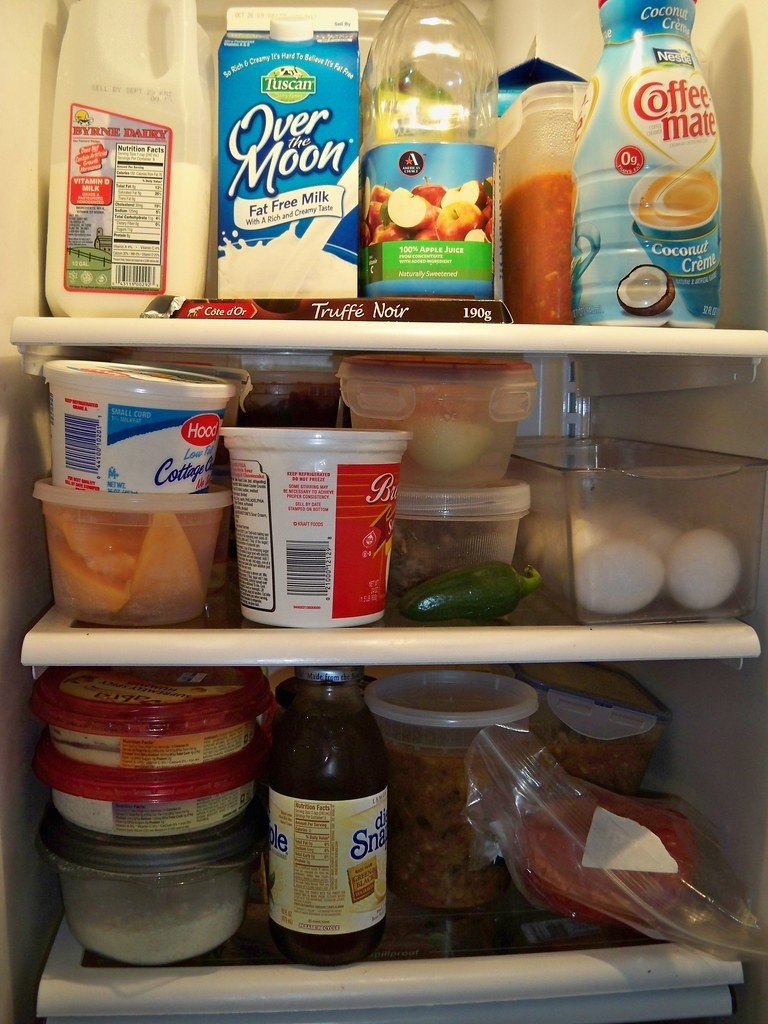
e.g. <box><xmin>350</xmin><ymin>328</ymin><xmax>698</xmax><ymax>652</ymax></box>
<box><xmin>362</xmin><ymin>173</ymin><xmax>496</xmax><ymax>246</ymax></box>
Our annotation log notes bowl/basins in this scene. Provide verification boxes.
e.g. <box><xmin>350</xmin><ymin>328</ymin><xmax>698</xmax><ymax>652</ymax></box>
<box><xmin>29</xmin><ymin>669</ymin><xmax>277</xmax><ymax>967</ymax></box>
<box><xmin>31</xmin><ymin>354</ymin><xmax>767</xmax><ymax>627</ymax></box>
<box><xmin>507</xmin><ymin>666</ymin><xmax>674</xmax><ymax>797</ymax></box>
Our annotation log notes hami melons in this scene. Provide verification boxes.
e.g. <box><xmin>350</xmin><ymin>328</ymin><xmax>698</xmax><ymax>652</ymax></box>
<box><xmin>39</xmin><ymin>504</ymin><xmax>209</xmax><ymax>622</ymax></box>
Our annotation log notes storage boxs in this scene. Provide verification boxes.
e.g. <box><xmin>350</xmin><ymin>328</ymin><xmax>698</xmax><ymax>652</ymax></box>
<box><xmin>507</xmin><ymin>435</ymin><xmax>767</xmax><ymax>626</ymax></box>
<box><xmin>216</xmin><ymin>6</ymin><xmax>361</xmax><ymax>301</ymax></box>
<box><xmin>333</xmin><ymin>356</ymin><xmax>540</xmax><ymax>489</ymax></box>
<box><xmin>510</xmin><ymin>663</ymin><xmax>673</xmax><ymax>793</ymax></box>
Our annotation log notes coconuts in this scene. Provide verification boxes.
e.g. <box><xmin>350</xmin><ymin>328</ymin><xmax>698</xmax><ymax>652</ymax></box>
<box><xmin>616</xmin><ymin>264</ymin><xmax>676</xmax><ymax>316</ymax></box>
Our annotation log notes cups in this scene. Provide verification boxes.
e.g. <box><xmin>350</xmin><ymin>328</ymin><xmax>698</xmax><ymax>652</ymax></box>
<box><xmin>363</xmin><ymin>673</ymin><xmax>539</xmax><ymax>908</ymax></box>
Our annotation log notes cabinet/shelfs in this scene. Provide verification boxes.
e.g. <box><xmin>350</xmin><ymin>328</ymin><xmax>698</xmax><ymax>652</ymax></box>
<box><xmin>0</xmin><ymin>319</ymin><xmax>768</xmax><ymax>1024</ymax></box>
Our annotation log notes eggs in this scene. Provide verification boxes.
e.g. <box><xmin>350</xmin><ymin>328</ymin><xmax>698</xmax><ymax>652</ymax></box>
<box><xmin>510</xmin><ymin>501</ymin><xmax>740</xmax><ymax>614</ymax></box>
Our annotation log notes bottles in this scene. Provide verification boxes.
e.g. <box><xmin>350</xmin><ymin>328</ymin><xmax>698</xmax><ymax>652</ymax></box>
<box><xmin>262</xmin><ymin>664</ymin><xmax>391</xmax><ymax>963</ymax></box>
<box><xmin>43</xmin><ymin>0</ymin><xmax>724</xmax><ymax>328</ymax></box>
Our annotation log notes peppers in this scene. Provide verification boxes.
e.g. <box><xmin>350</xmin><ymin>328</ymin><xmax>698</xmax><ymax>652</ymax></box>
<box><xmin>397</xmin><ymin>560</ymin><xmax>542</xmax><ymax>621</ymax></box>
